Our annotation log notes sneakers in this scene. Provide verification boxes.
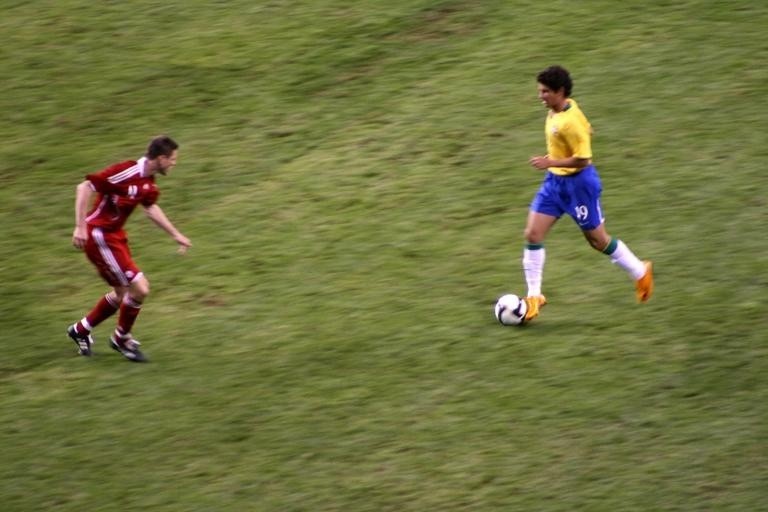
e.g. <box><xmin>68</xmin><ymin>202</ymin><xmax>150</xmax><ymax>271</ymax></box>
<box><xmin>67</xmin><ymin>324</ymin><xmax>90</xmax><ymax>356</ymax></box>
<box><xmin>525</xmin><ymin>295</ymin><xmax>546</xmax><ymax>320</ymax></box>
<box><xmin>636</xmin><ymin>261</ymin><xmax>652</xmax><ymax>301</ymax></box>
<box><xmin>110</xmin><ymin>333</ymin><xmax>144</xmax><ymax>362</ymax></box>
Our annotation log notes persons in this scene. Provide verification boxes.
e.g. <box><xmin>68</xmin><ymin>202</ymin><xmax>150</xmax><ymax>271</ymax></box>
<box><xmin>516</xmin><ymin>64</ymin><xmax>654</xmax><ymax>320</ymax></box>
<box><xmin>64</xmin><ymin>136</ymin><xmax>192</xmax><ymax>364</ymax></box>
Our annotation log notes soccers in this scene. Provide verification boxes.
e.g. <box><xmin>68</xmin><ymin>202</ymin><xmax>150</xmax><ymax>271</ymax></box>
<box><xmin>493</xmin><ymin>294</ymin><xmax>526</xmax><ymax>326</ymax></box>
